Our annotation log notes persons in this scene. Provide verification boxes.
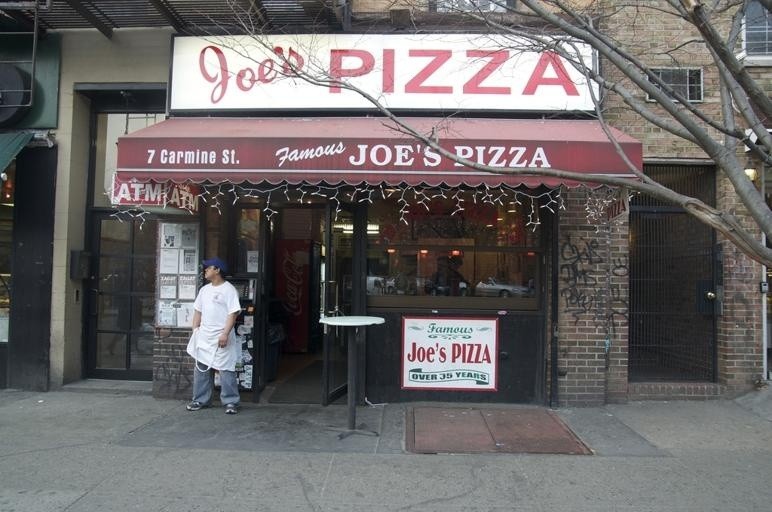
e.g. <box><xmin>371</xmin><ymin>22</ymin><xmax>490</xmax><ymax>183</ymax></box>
<box><xmin>186</xmin><ymin>257</ymin><xmax>242</xmax><ymax>414</ymax></box>
<box><xmin>424</xmin><ymin>255</ymin><xmax>469</xmax><ymax>296</ymax></box>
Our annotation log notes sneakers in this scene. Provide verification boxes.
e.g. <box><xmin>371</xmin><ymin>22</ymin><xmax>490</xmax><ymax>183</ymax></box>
<box><xmin>186</xmin><ymin>400</ymin><xmax>213</xmax><ymax>411</ymax></box>
<box><xmin>224</xmin><ymin>401</ymin><xmax>239</xmax><ymax>414</ymax></box>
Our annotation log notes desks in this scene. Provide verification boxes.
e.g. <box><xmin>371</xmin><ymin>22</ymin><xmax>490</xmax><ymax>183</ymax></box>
<box><xmin>320</xmin><ymin>313</ymin><xmax>385</xmax><ymax>437</ymax></box>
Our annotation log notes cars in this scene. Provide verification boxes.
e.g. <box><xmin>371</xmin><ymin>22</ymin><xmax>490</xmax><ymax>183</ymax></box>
<box><xmin>368</xmin><ymin>275</ymin><xmax>532</xmax><ymax>298</ymax></box>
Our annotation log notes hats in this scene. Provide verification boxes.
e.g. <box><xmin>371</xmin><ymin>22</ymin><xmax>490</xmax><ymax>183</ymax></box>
<box><xmin>201</xmin><ymin>257</ymin><xmax>226</xmax><ymax>272</ymax></box>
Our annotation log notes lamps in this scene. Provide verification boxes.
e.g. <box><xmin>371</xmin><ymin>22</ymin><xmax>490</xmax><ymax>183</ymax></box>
<box><xmin>743</xmin><ymin>159</ymin><xmax>759</xmax><ymax>190</ymax></box>
